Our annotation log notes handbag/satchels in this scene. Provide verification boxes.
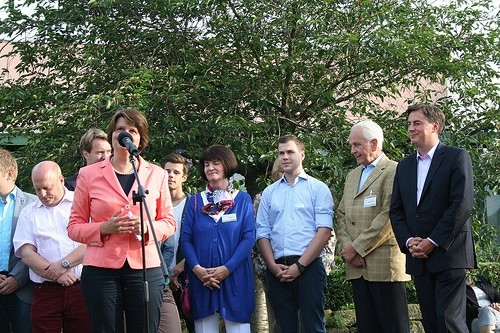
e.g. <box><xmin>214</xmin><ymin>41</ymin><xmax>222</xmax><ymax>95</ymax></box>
<box><xmin>180</xmin><ymin>287</ymin><xmax>191</xmax><ymax>318</ymax></box>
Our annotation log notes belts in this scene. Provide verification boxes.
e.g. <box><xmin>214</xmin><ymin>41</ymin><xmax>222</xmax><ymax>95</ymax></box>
<box><xmin>45</xmin><ymin>281</ymin><xmax>60</xmax><ymax>286</ymax></box>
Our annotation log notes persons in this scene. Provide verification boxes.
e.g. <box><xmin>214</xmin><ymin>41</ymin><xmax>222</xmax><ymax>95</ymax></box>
<box><xmin>389</xmin><ymin>103</ymin><xmax>477</xmax><ymax>333</ymax></box>
<box><xmin>0</xmin><ymin>109</ymin><xmax>334</xmax><ymax>333</ymax></box>
<box><xmin>466</xmin><ymin>280</ymin><xmax>500</xmax><ymax>333</ymax></box>
<box><xmin>336</xmin><ymin>120</ymin><xmax>412</xmax><ymax>333</ymax></box>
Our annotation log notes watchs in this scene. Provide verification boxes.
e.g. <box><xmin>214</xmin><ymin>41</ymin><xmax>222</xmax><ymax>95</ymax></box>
<box><xmin>61</xmin><ymin>259</ymin><xmax>69</xmax><ymax>270</ymax></box>
<box><xmin>295</xmin><ymin>261</ymin><xmax>305</xmax><ymax>273</ymax></box>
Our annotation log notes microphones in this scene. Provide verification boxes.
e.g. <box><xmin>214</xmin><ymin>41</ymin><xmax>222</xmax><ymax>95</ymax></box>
<box><xmin>117</xmin><ymin>132</ymin><xmax>139</xmax><ymax>157</ymax></box>
